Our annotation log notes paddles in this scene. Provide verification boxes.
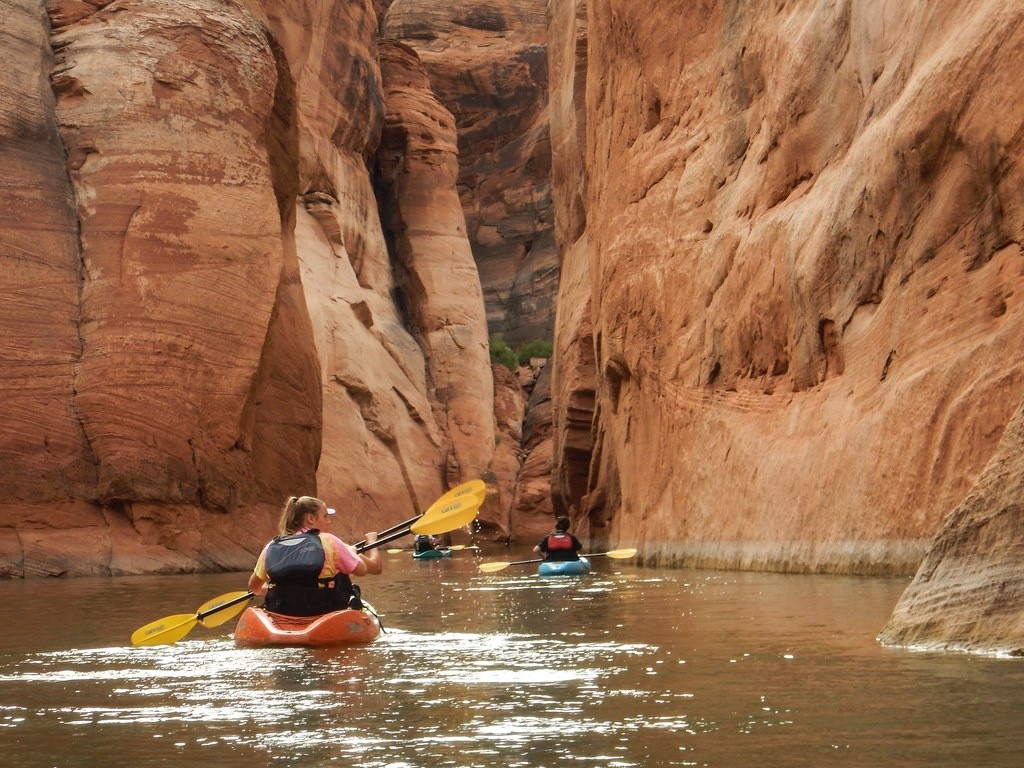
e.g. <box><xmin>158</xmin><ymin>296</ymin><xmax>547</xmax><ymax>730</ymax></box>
<box><xmin>129</xmin><ymin>492</ymin><xmax>481</xmax><ymax>650</ymax></box>
<box><xmin>477</xmin><ymin>547</ymin><xmax>641</xmax><ymax>575</ymax></box>
<box><xmin>390</xmin><ymin>542</ymin><xmax>481</xmax><ymax>557</ymax></box>
<box><xmin>194</xmin><ymin>474</ymin><xmax>490</xmax><ymax>630</ymax></box>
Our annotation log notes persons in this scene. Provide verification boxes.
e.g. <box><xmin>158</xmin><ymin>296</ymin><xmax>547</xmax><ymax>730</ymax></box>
<box><xmin>411</xmin><ymin>534</ymin><xmax>441</xmax><ymax>554</ymax></box>
<box><xmin>249</xmin><ymin>495</ymin><xmax>382</xmax><ymax>617</ymax></box>
<box><xmin>533</xmin><ymin>516</ymin><xmax>586</xmax><ymax>562</ymax></box>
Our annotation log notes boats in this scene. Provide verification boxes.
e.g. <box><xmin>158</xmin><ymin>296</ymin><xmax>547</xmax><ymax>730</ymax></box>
<box><xmin>234</xmin><ymin>597</ymin><xmax>381</xmax><ymax>645</ymax></box>
<box><xmin>538</xmin><ymin>553</ymin><xmax>591</xmax><ymax>575</ymax></box>
<box><xmin>412</xmin><ymin>547</ymin><xmax>452</xmax><ymax>558</ymax></box>
